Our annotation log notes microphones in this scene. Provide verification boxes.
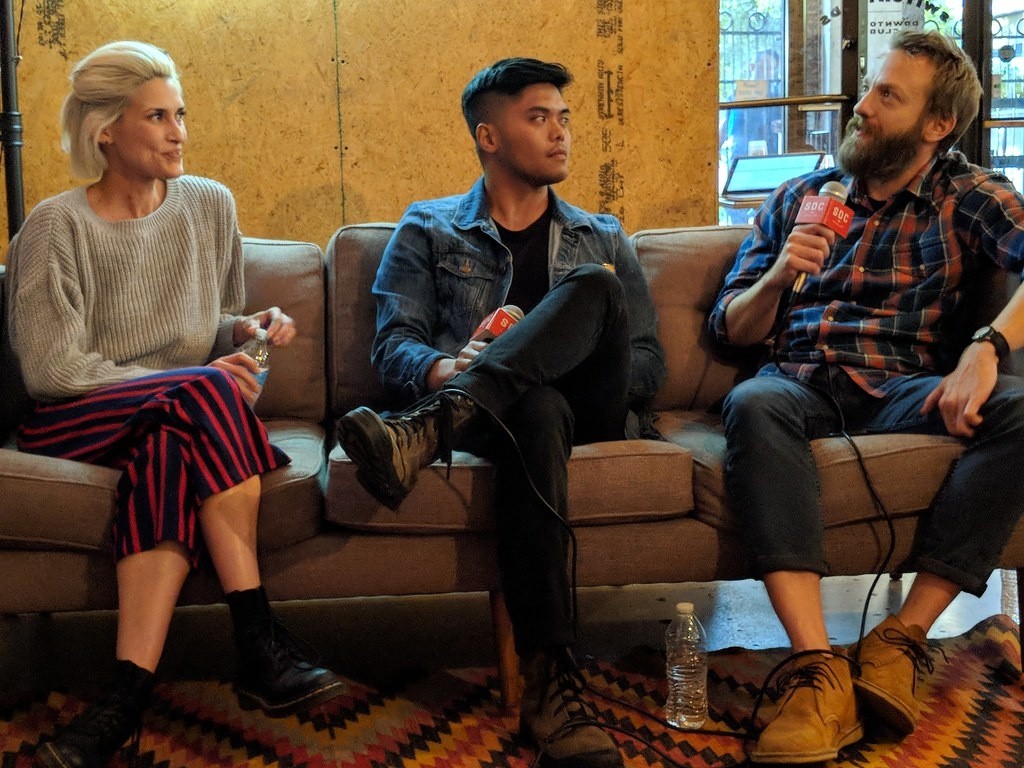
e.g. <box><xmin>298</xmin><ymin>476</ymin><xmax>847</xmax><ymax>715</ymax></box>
<box><xmin>469</xmin><ymin>305</ymin><xmax>524</xmax><ymax>345</ymax></box>
<box><xmin>789</xmin><ymin>182</ymin><xmax>855</xmax><ymax>305</ymax></box>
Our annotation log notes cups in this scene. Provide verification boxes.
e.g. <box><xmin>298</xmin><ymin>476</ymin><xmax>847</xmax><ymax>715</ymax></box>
<box><xmin>811</xmin><ymin>130</ymin><xmax>831</xmax><ymax>155</ymax></box>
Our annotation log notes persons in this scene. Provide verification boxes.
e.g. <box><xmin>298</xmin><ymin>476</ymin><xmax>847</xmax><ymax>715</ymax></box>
<box><xmin>705</xmin><ymin>37</ymin><xmax>1024</xmax><ymax>768</ymax></box>
<box><xmin>7</xmin><ymin>41</ymin><xmax>346</xmax><ymax>747</ymax></box>
<box><xmin>340</xmin><ymin>59</ymin><xmax>669</xmax><ymax>768</ymax></box>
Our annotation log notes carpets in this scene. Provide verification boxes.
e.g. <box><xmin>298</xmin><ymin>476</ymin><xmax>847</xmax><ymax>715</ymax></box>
<box><xmin>0</xmin><ymin>612</ymin><xmax>1024</xmax><ymax>768</ymax></box>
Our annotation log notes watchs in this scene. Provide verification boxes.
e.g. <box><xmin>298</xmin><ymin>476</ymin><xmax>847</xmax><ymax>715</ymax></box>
<box><xmin>971</xmin><ymin>327</ymin><xmax>1011</xmax><ymax>362</ymax></box>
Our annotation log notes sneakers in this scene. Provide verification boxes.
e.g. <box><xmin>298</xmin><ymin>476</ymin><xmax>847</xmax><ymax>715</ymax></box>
<box><xmin>337</xmin><ymin>392</ymin><xmax>458</xmax><ymax>512</ymax></box>
<box><xmin>517</xmin><ymin>657</ymin><xmax>625</xmax><ymax>768</ymax></box>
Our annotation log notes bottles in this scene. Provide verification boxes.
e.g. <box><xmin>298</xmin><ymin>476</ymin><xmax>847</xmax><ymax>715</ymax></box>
<box><xmin>234</xmin><ymin>326</ymin><xmax>272</xmax><ymax>406</ymax></box>
<box><xmin>748</xmin><ymin>140</ymin><xmax>768</xmax><ymax>155</ymax></box>
<box><xmin>663</xmin><ymin>600</ymin><xmax>709</xmax><ymax>731</ymax></box>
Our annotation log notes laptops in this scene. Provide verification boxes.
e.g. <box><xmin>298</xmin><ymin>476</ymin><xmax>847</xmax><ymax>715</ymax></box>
<box><xmin>720</xmin><ymin>151</ymin><xmax>826</xmax><ymax>202</ymax></box>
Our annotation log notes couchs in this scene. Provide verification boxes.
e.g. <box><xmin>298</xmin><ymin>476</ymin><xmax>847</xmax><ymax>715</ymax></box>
<box><xmin>0</xmin><ymin>223</ymin><xmax>1024</xmax><ymax>705</ymax></box>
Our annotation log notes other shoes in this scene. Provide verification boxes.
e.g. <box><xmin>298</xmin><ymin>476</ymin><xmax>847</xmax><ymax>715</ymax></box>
<box><xmin>848</xmin><ymin>614</ymin><xmax>949</xmax><ymax>736</ymax></box>
<box><xmin>753</xmin><ymin>645</ymin><xmax>867</xmax><ymax>764</ymax></box>
<box><xmin>36</xmin><ymin>661</ymin><xmax>154</xmax><ymax>768</ymax></box>
<box><xmin>227</xmin><ymin>585</ymin><xmax>344</xmax><ymax>719</ymax></box>
